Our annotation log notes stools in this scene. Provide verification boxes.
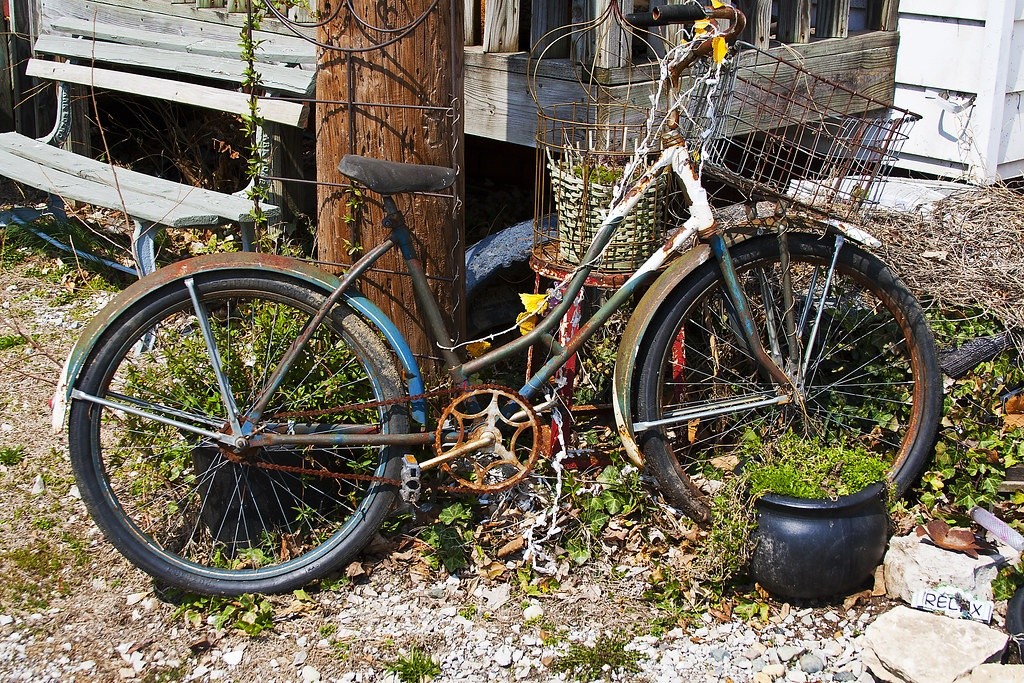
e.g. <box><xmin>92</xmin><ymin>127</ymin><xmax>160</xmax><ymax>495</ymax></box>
<box><xmin>524</xmin><ymin>239</ymin><xmax>686</xmax><ymax>483</ymax></box>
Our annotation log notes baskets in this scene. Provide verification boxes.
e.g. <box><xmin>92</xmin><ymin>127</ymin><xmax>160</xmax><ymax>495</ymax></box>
<box><xmin>678</xmin><ymin>40</ymin><xmax>923</xmax><ymax>228</ymax></box>
<box><xmin>546</xmin><ymin>126</ymin><xmax>667</xmax><ymax>269</ymax></box>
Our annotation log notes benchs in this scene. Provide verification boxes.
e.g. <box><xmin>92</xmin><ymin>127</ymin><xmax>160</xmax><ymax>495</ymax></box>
<box><xmin>0</xmin><ymin>17</ymin><xmax>320</xmax><ymax>358</ymax></box>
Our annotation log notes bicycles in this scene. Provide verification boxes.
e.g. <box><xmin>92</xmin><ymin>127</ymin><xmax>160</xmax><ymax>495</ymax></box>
<box><xmin>47</xmin><ymin>2</ymin><xmax>947</xmax><ymax>602</ymax></box>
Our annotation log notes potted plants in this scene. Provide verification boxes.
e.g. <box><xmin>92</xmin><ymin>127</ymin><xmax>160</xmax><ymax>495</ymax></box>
<box><xmin>546</xmin><ymin>126</ymin><xmax>667</xmax><ymax>271</ymax></box>
<box><xmin>686</xmin><ymin>424</ymin><xmax>910</xmax><ymax>602</ymax></box>
